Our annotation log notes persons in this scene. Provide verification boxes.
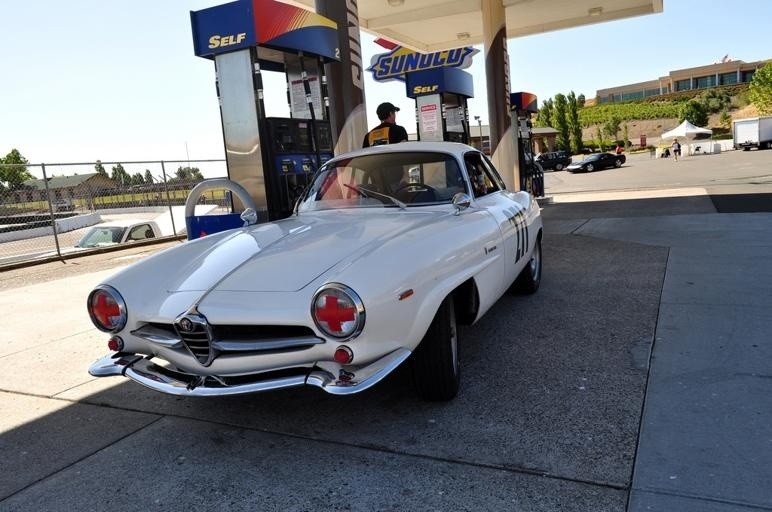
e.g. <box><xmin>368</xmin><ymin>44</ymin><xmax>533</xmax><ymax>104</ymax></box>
<box><xmin>363</xmin><ymin>102</ymin><xmax>410</xmax><ymax>194</ymax></box>
<box><xmin>616</xmin><ymin>144</ymin><xmax>624</xmax><ymax>154</ymax></box>
<box><xmin>672</xmin><ymin>138</ymin><xmax>681</xmax><ymax>161</ymax></box>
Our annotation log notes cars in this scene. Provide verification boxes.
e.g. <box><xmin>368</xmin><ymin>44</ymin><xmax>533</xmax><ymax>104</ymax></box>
<box><xmin>33</xmin><ymin>219</ymin><xmax>162</xmax><ymax>258</ymax></box>
<box><xmin>533</xmin><ymin>151</ymin><xmax>626</xmax><ymax>173</ymax></box>
<box><xmin>87</xmin><ymin>141</ymin><xmax>544</xmax><ymax>402</ymax></box>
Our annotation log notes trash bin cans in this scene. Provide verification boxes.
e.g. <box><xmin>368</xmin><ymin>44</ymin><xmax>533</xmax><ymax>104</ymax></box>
<box><xmin>714</xmin><ymin>144</ymin><xmax>721</xmax><ymax>153</ymax></box>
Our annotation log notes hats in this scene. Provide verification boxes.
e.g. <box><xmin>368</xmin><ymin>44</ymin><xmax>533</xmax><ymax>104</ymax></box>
<box><xmin>376</xmin><ymin>102</ymin><xmax>400</xmax><ymax>118</ymax></box>
